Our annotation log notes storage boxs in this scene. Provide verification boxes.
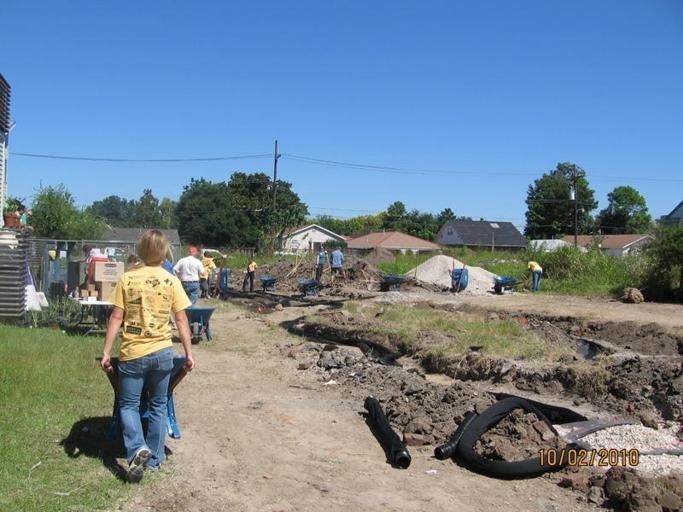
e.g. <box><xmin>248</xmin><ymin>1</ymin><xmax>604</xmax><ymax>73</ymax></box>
<box><xmin>76</xmin><ymin>259</ymin><xmax>127</xmax><ymax>301</ymax></box>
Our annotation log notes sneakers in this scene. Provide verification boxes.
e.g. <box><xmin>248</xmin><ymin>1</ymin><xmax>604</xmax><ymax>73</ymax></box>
<box><xmin>125</xmin><ymin>447</ymin><xmax>152</xmax><ymax>483</ymax></box>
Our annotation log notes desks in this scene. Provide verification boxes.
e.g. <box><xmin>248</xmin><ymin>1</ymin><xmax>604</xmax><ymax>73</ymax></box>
<box><xmin>73</xmin><ymin>297</ymin><xmax>115</xmax><ymax>336</ymax></box>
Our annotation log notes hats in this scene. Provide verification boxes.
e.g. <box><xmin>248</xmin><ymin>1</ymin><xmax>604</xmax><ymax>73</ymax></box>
<box><xmin>188</xmin><ymin>247</ymin><xmax>198</xmax><ymax>253</ymax></box>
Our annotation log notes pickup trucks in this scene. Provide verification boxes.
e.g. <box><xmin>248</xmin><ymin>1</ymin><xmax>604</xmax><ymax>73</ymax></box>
<box><xmin>174</xmin><ymin>248</ymin><xmax>230</xmax><ymax>262</ymax></box>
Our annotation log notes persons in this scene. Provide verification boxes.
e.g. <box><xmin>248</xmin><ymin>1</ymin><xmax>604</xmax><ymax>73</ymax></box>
<box><xmin>526</xmin><ymin>260</ymin><xmax>542</xmax><ymax>293</ymax></box>
<box><xmin>80</xmin><ymin>243</ymin><xmax>106</xmax><ymax>277</ymax></box>
<box><xmin>174</xmin><ymin>246</ymin><xmax>205</xmax><ymax>307</ymax></box>
<box><xmin>13</xmin><ymin>207</ymin><xmax>34</xmax><ymax>232</ymax></box>
<box><xmin>99</xmin><ymin>229</ymin><xmax>196</xmax><ymax>484</ymax></box>
<box><xmin>126</xmin><ymin>254</ymin><xmax>141</xmax><ymax>268</ymax></box>
<box><xmin>198</xmin><ymin>250</ymin><xmax>216</xmax><ymax>300</ymax></box>
<box><xmin>329</xmin><ymin>246</ymin><xmax>347</xmax><ymax>287</ymax></box>
<box><xmin>314</xmin><ymin>246</ymin><xmax>331</xmax><ymax>281</ymax></box>
<box><xmin>160</xmin><ymin>258</ymin><xmax>174</xmax><ymax>274</ymax></box>
<box><xmin>238</xmin><ymin>257</ymin><xmax>257</xmax><ymax>292</ymax></box>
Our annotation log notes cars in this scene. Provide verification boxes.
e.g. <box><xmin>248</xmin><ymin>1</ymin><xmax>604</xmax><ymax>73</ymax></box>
<box><xmin>98</xmin><ymin>246</ymin><xmax>127</xmax><ymax>257</ymax></box>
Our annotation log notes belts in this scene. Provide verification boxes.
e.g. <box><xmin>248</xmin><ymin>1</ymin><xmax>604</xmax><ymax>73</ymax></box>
<box><xmin>181</xmin><ymin>281</ymin><xmax>198</xmax><ymax>283</ymax></box>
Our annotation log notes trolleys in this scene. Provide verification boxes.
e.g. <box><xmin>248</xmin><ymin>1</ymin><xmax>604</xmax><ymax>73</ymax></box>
<box><xmin>448</xmin><ymin>257</ymin><xmax>471</xmax><ymax>294</ymax></box>
<box><xmin>92</xmin><ymin>354</ymin><xmax>192</xmax><ymax>441</ymax></box>
<box><xmin>183</xmin><ymin>305</ymin><xmax>217</xmax><ymax>345</ymax></box>
<box><xmin>380</xmin><ymin>273</ymin><xmax>411</xmax><ymax>292</ymax></box>
<box><xmin>260</xmin><ymin>276</ymin><xmax>278</xmax><ymax>292</ymax></box>
<box><xmin>490</xmin><ymin>276</ymin><xmax>525</xmax><ymax>295</ymax></box>
<box><xmin>297</xmin><ymin>278</ymin><xmax>320</xmax><ymax>298</ymax></box>
